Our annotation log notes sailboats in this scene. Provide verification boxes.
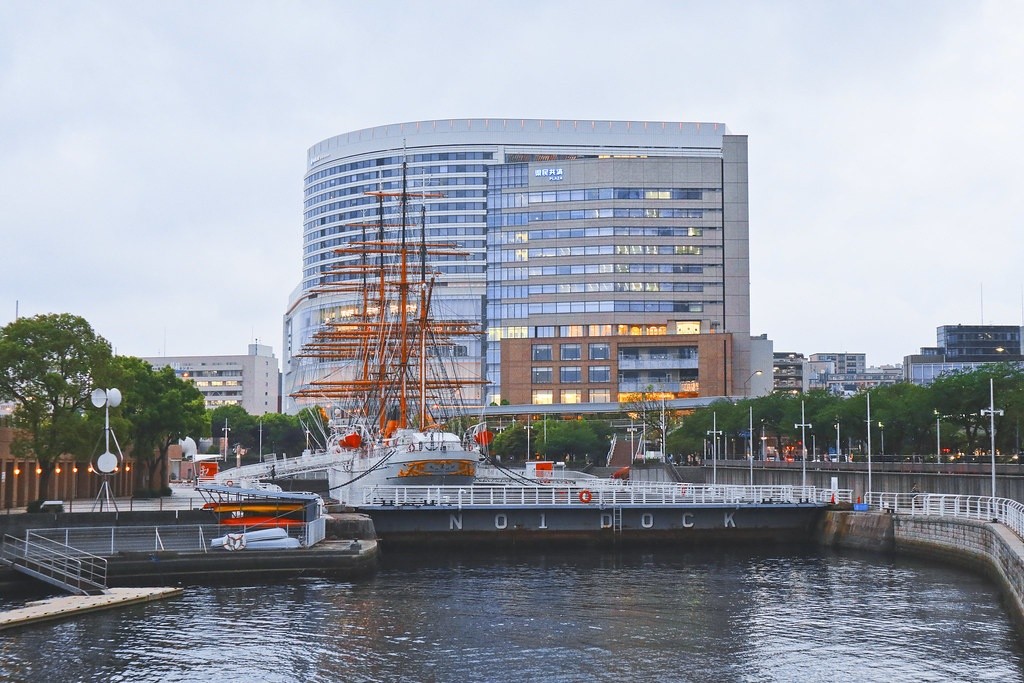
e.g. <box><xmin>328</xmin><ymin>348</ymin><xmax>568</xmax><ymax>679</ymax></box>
<box><xmin>289</xmin><ymin>137</ymin><xmax>495</xmax><ymax>503</ymax></box>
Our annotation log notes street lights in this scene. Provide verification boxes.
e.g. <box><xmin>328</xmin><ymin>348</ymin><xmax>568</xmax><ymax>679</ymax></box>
<box><xmin>744</xmin><ymin>371</ymin><xmax>762</xmax><ymax>399</ymax></box>
<box><xmin>834</xmin><ymin>422</ymin><xmax>840</xmax><ymax>462</ymax></box>
<box><xmin>996</xmin><ymin>347</ymin><xmax>1019</xmax><ymax>463</ymax></box>
<box><xmin>879</xmin><ymin>422</ymin><xmax>885</xmax><ymax>455</ymax></box>
<box><xmin>933</xmin><ymin>409</ymin><xmax>943</xmax><ymax>463</ymax></box>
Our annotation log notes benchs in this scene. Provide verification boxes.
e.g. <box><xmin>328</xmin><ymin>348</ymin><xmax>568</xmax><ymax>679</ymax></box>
<box><xmin>882</xmin><ymin>508</ymin><xmax>899</xmax><ymax>514</ymax></box>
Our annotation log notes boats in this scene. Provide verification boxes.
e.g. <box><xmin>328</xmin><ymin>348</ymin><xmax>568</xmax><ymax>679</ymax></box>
<box><xmin>215</xmin><ymin>536</ymin><xmax>301</xmax><ymax>550</ymax></box>
<box><xmin>211</xmin><ymin>527</ymin><xmax>287</xmax><ymax>547</ymax></box>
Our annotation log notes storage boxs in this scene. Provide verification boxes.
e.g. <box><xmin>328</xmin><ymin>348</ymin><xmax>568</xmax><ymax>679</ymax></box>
<box><xmin>853</xmin><ymin>503</ymin><xmax>869</xmax><ymax>511</ymax></box>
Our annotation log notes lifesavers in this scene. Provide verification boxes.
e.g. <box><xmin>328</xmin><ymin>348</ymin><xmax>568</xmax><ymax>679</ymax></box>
<box><xmin>469</xmin><ymin>444</ymin><xmax>473</xmax><ymax>451</ymax></box>
<box><xmin>579</xmin><ymin>490</ymin><xmax>592</xmax><ymax>502</ymax></box>
<box><xmin>409</xmin><ymin>444</ymin><xmax>415</xmax><ymax>452</ymax></box>
<box><xmin>226</xmin><ymin>481</ymin><xmax>233</xmax><ymax>487</ymax></box>
<box><xmin>230</xmin><ymin>533</ymin><xmax>247</xmax><ymax>550</ymax></box>
<box><xmin>223</xmin><ymin>533</ymin><xmax>240</xmax><ymax>551</ymax></box>
